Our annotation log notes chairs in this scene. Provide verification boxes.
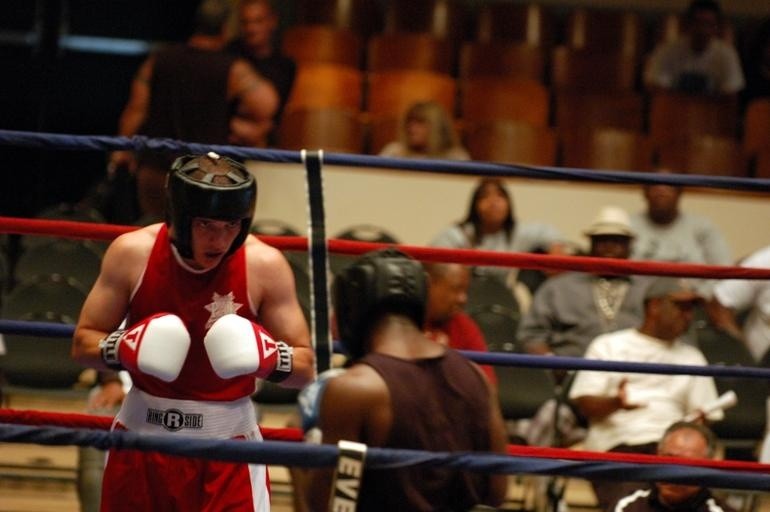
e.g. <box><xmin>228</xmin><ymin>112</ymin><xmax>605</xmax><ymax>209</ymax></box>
<box><xmin>3</xmin><ymin>209</ymin><xmax>397</xmax><ymax>417</ymax></box>
<box><xmin>110</xmin><ymin>0</ymin><xmax>770</xmax><ymax>186</ymax></box>
<box><xmin>397</xmin><ymin>223</ymin><xmax>770</xmax><ymax>466</ymax></box>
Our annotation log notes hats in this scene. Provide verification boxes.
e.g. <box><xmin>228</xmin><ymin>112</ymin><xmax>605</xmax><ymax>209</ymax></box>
<box><xmin>643</xmin><ymin>276</ymin><xmax>707</xmax><ymax>308</ymax></box>
<box><xmin>587</xmin><ymin>206</ymin><xmax>635</xmax><ymax>238</ymax></box>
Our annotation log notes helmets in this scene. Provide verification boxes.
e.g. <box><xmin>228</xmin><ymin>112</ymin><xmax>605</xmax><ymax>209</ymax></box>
<box><xmin>332</xmin><ymin>254</ymin><xmax>428</xmax><ymax>351</ymax></box>
<box><xmin>164</xmin><ymin>154</ymin><xmax>256</xmax><ymax>259</ymax></box>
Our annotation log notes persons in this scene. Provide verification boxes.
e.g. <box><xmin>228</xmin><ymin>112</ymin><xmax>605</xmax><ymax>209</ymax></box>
<box><xmin>2</xmin><ymin>3</ymin><xmax>75</xmax><ymax>216</ymax></box>
<box><xmin>75</xmin><ymin>2</ymin><xmax>768</xmax><ymax>512</ymax></box>
<box><xmin>71</xmin><ymin>150</ymin><xmax>316</xmax><ymax>512</ymax></box>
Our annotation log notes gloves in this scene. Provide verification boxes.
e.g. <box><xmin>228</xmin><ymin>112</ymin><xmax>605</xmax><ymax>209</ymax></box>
<box><xmin>101</xmin><ymin>312</ymin><xmax>193</xmax><ymax>383</ymax></box>
<box><xmin>204</xmin><ymin>313</ymin><xmax>293</xmax><ymax>380</ymax></box>
